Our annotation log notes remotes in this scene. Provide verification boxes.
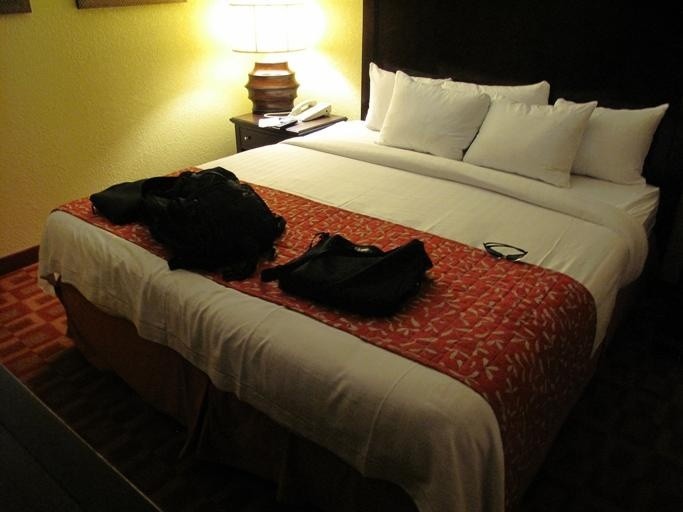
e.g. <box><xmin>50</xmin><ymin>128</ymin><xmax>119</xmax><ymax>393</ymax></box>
<box><xmin>271</xmin><ymin>116</ymin><xmax>298</xmax><ymax>130</ymax></box>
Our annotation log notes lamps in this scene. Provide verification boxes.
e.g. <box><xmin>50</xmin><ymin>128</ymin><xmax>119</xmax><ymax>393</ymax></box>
<box><xmin>227</xmin><ymin>2</ymin><xmax>306</xmax><ymax>118</ymax></box>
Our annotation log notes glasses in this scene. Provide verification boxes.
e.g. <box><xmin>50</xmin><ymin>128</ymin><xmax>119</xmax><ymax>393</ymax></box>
<box><xmin>482</xmin><ymin>241</ymin><xmax>528</xmax><ymax>261</ymax></box>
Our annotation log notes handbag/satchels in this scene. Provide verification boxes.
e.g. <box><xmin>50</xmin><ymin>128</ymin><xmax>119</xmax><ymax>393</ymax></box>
<box><xmin>257</xmin><ymin>230</ymin><xmax>435</xmax><ymax>318</ymax></box>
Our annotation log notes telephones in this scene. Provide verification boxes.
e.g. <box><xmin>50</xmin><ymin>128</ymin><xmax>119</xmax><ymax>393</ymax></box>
<box><xmin>288</xmin><ymin>99</ymin><xmax>332</xmax><ymax>122</ymax></box>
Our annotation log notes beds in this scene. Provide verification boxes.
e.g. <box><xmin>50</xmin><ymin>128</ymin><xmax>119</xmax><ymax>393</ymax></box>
<box><xmin>40</xmin><ymin>1</ymin><xmax>682</xmax><ymax>512</ymax></box>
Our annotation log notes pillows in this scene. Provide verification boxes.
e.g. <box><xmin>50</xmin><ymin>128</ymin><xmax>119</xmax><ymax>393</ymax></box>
<box><xmin>444</xmin><ymin>80</ymin><xmax>550</xmax><ymax>104</ymax></box>
<box><xmin>463</xmin><ymin>101</ymin><xmax>599</xmax><ymax>189</ymax></box>
<box><xmin>554</xmin><ymin>97</ymin><xmax>669</xmax><ymax>185</ymax></box>
<box><xmin>375</xmin><ymin>70</ymin><xmax>491</xmax><ymax>161</ymax></box>
<box><xmin>363</xmin><ymin>62</ymin><xmax>452</xmax><ymax>131</ymax></box>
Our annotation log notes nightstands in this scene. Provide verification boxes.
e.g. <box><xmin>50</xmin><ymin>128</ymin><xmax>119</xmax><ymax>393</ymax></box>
<box><xmin>230</xmin><ymin>114</ymin><xmax>347</xmax><ymax>155</ymax></box>
